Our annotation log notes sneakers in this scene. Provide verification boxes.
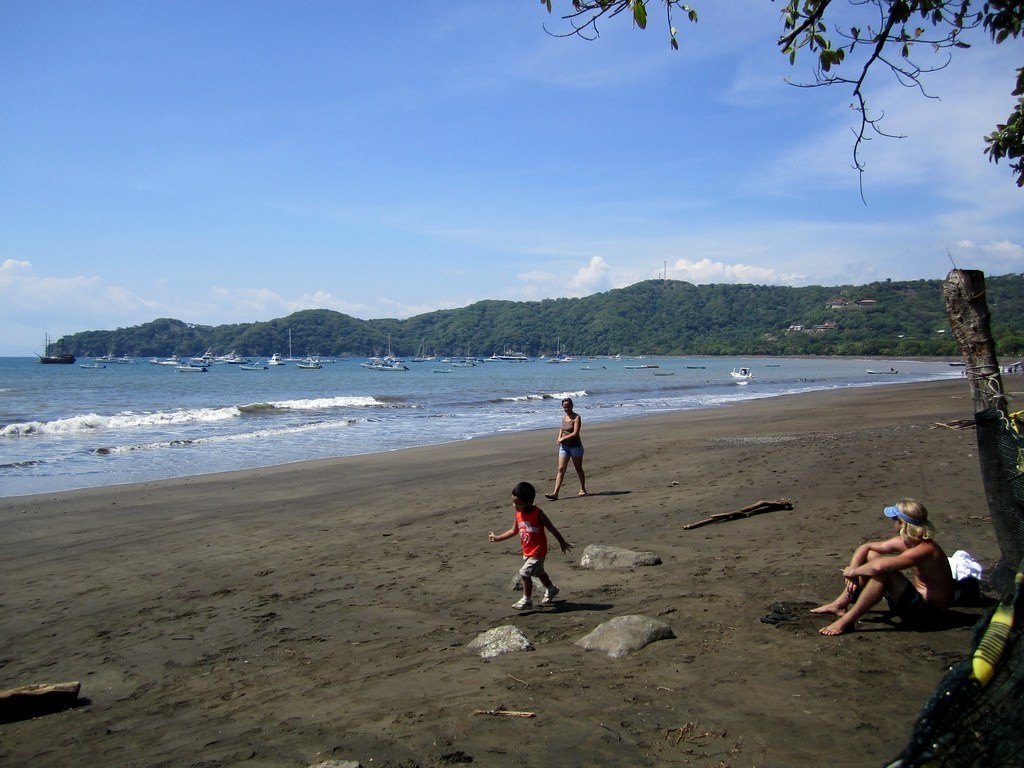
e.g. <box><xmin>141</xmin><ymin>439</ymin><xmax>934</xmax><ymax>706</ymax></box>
<box><xmin>542</xmin><ymin>584</ymin><xmax>560</xmax><ymax>603</ymax></box>
<box><xmin>511</xmin><ymin>599</ymin><xmax>533</xmax><ymax>610</ymax></box>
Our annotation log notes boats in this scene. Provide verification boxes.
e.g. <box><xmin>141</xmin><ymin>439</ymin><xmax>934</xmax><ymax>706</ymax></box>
<box><xmin>300</xmin><ymin>356</ymin><xmax>337</xmax><ymax>365</ymax></box>
<box><xmin>432</xmin><ymin>368</ymin><xmax>453</xmax><ymax>373</ymax></box>
<box><xmin>175</xmin><ymin>364</ymin><xmax>206</xmax><ymax>372</ymax></box>
<box><xmin>93</xmin><ymin>352</ymin><xmax>117</xmax><ymax>363</ymax></box>
<box><xmin>189</xmin><ymin>361</ymin><xmax>212</xmax><ymax>367</ymax></box>
<box><xmin>297</xmin><ymin>362</ymin><xmax>320</xmax><ymax>369</ymax></box>
<box><xmin>547</xmin><ymin>358</ymin><xmax>565</xmax><ymax>364</ymax></box>
<box><xmin>159</xmin><ymin>355</ymin><xmax>187</xmax><ymax>366</ymax></box>
<box><xmin>360</xmin><ymin>355</ymin><xmax>410</xmax><ymax>371</ymax></box>
<box><xmin>624</xmin><ymin>364</ymin><xmax>660</xmax><ymax>369</ymax></box>
<box><xmin>948</xmin><ymin>363</ymin><xmax>966</xmax><ymax>366</ymax></box>
<box><xmin>369</xmin><ymin>356</ymin><xmax>380</xmax><ymax>359</ymax></box>
<box><xmin>225</xmin><ymin>357</ymin><xmax>248</xmax><ymax>365</ymax></box>
<box><xmin>117</xmin><ymin>353</ymin><xmax>138</xmax><ymax>364</ymax></box>
<box><xmin>654</xmin><ymin>371</ymin><xmax>674</xmax><ymax>376</ymax></box>
<box><xmin>443</xmin><ymin>340</ymin><xmax>530</xmax><ymax>367</ymax></box>
<box><xmin>148</xmin><ymin>357</ymin><xmax>163</xmax><ymax>364</ymax></box>
<box><xmin>190</xmin><ymin>355</ymin><xmax>215</xmax><ymax>364</ymax></box>
<box><xmin>41</xmin><ymin>354</ymin><xmax>76</xmax><ymax>364</ymax></box>
<box><xmin>239</xmin><ymin>363</ymin><xmax>269</xmax><ymax>371</ymax></box>
<box><xmin>267</xmin><ymin>353</ymin><xmax>286</xmax><ymax>365</ymax></box>
<box><xmin>730</xmin><ymin>367</ymin><xmax>755</xmax><ymax>379</ymax></box>
<box><xmin>580</xmin><ymin>366</ymin><xmax>606</xmax><ymax>370</ymax></box>
<box><xmin>215</xmin><ymin>349</ymin><xmax>237</xmax><ymax>361</ymax></box>
<box><xmin>79</xmin><ymin>363</ymin><xmax>106</xmax><ymax>369</ymax></box>
<box><xmin>562</xmin><ymin>357</ymin><xmax>577</xmax><ymax>363</ymax></box>
<box><xmin>685</xmin><ymin>365</ymin><xmax>707</xmax><ymax>369</ymax></box>
<box><xmin>867</xmin><ymin>368</ymin><xmax>898</xmax><ymax>374</ymax></box>
<box><xmin>539</xmin><ymin>355</ymin><xmax>549</xmax><ymax>360</ymax></box>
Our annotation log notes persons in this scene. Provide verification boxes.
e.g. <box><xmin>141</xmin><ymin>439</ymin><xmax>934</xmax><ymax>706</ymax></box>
<box><xmin>810</xmin><ymin>498</ymin><xmax>953</xmax><ymax>636</ymax></box>
<box><xmin>1002</xmin><ymin>365</ymin><xmax>1022</xmax><ymax>377</ymax></box>
<box><xmin>489</xmin><ymin>481</ymin><xmax>574</xmax><ymax>610</ymax></box>
<box><xmin>545</xmin><ymin>397</ymin><xmax>587</xmax><ymax>499</ymax></box>
<box><xmin>961</xmin><ymin>369</ymin><xmax>965</xmax><ymax>378</ymax></box>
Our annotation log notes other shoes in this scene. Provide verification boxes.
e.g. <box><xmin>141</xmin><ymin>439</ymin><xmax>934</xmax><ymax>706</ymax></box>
<box><xmin>546</xmin><ymin>493</ymin><xmax>559</xmax><ymax>500</ymax></box>
<box><xmin>577</xmin><ymin>490</ymin><xmax>586</xmax><ymax>497</ymax></box>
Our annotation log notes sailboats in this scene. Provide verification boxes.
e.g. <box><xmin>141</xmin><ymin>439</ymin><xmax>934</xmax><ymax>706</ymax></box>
<box><xmin>409</xmin><ymin>338</ymin><xmax>439</xmax><ymax>362</ymax></box>
<box><xmin>282</xmin><ymin>329</ymin><xmax>301</xmax><ymax>362</ymax></box>
<box><xmin>380</xmin><ymin>333</ymin><xmax>406</xmax><ymax>363</ymax></box>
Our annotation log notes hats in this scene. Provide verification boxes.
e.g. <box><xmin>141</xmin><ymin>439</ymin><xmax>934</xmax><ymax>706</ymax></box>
<box><xmin>883</xmin><ymin>503</ymin><xmax>927</xmax><ymax>525</ymax></box>
<box><xmin>947</xmin><ymin>549</ymin><xmax>983</xmax><ymax>581</ymax></box>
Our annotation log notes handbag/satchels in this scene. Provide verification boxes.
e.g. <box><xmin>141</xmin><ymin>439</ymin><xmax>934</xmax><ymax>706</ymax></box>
<box><xmin>953</xmin><ymin>576</ymin><xmax>980</xmax><ymax>607</ymax></box>
